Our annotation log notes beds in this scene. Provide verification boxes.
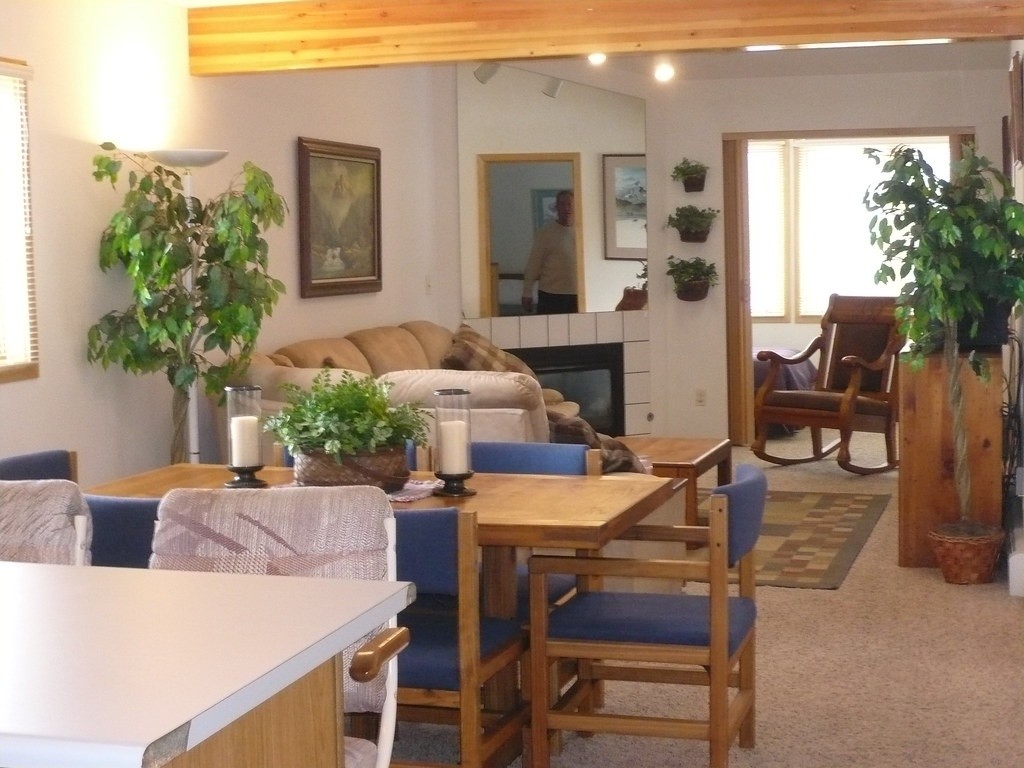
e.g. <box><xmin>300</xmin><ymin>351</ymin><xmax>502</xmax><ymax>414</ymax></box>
<box><xmin>753</xmin><ymin>346</ymin><xmax>817</xmax><ymax>439</ymax></box>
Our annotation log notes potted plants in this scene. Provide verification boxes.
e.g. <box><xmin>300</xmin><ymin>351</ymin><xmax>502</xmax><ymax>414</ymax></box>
<box><xmin>671</xmin><ymin>156</ymin><xmax>709</xmax><ymax>192</ymax></box>
<box><xmin>661</xmin><ymin>206</ymin><xmax>720</xmax><ymax>243</ymax></box>
<box><xmin>861</xmin><ymin>140</ymin><xmax>1024</xmax><ymax>585</ymax></box>
<box><xmin>667</xmin><ymin>254</ymin><xmax>718</xmax><ymax>302</ymax></box>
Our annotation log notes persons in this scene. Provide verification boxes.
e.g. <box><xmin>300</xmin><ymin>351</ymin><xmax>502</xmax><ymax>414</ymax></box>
<box><xmin>521</xmin><ymin>192</ymin><xmax>579</xmax><ymax>315</ymax></box>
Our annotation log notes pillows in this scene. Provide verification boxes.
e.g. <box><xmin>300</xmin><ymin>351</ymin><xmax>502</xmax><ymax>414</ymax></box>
<box><xmin>439</xmin><ymin>321</ymin><xmax>539</xmax><ymax>384</ymax></box>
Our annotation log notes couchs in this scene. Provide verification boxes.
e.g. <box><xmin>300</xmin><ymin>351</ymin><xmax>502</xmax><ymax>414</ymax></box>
<box><xmin>209</xmin><ymin>319</ymin><xmax>687</xmax><ymax>596</ymax></box>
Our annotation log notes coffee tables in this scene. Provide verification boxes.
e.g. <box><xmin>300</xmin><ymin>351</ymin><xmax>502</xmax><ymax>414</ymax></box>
<box><xmin>615</xmin><ymin>434</ymin><xmax>733</xmax><ymax>549</ymax></box>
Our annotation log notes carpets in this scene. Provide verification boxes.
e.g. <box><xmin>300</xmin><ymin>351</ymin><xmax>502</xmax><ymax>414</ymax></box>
<box><xmin>685</xmin><ymin>487</ymin><xmax>892</xmax><ymax>590</ymax></box>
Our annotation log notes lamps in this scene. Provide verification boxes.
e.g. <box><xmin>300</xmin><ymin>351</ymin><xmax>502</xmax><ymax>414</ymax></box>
<box><xmin>141</xmin><ymin>148</ymin><xmax>228</xmax><ymax>464</ymax></box>
<box><xmin>473</xmin><ymin>62</ymin><xmax>502</xmax><ymax>85</ymax></box>
<box><xmin>542</xmin><ymin>77</ymin><xmax>562</xmax><ymax>100</ymax></box>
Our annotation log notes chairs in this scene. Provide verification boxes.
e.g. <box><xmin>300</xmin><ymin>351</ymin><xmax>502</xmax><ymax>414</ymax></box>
<box><xmin>0</xmin><ymin>449</ymin><xmax>78</xmax><ymax>485</ymax></box>
<box><xmin>80</xmin><ymin>493</ymin><xmax>163</xmax><ymax>568</ymax></box>
<box><xmin>274</xmin><ymin>441</ymin><xmax>432</xmax><ymax>473</ymax></box>
<box><xmin>348</xmin><ymin>507</ymin><xmax>525</xmax><ymax>768</ymax></box>
<box><xmin>0</xmin><ymin>479</ymin><xmax>93</xmax><ymax>566</ymax></box>
<box><xmin>750</xmin><ymin>291</ymin><xmax>911</xmax><ymax>474</ymax></box>
<box><xmin>527</xmin><ymin>465</ymin><xmax>769</xmax><ymax>768</ymax></box>
<box><xmin>416</xmin><ymin>443</ymin><xmax>603</xmax><ymax>756</ymax></box>
<box><xmin>148</xmin><ymin>485</ymin><xmax>410</xmax><ymax>768</ymax></box>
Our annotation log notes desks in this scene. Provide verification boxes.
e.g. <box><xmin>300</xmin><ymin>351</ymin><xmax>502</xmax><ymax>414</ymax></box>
<box><xmin>0</xmin><ymin>560</ymin><xmax>415</xmax><ymax>768</ymax></box>
<box><xmin>86</xmin><ymin>461</ymin><xmax>674</xmax><ymax>768</ymax></box>
<box><xmin>897</xmin><ymin>351</ymin><xmax>1002</xmax><ymax>570</ymax></box>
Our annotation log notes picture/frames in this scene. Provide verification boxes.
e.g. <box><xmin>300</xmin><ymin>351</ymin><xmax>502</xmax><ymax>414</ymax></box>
<box><xmin>1002</xmin><ymin>115</ymin><xmax>1011</xmax><ymax>196</ymax></box>
<box><xmin>1008</xmin><ymin>51</ymin><xmax>1024</xmax><ymax>170</ymax></box>
<box><xmin>298</xmin><ymin>136</ymin><xmax>383</xmax><ymax>299</ymax></box>
<box><xmin>602</xmin><ymin>154</ymin><xmax>647</xmax><ymax>261</ymax></box>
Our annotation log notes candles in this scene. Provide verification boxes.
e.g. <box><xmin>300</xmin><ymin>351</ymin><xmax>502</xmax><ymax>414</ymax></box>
<box><xmin>224</xmin><ymin>384</ymin><xmax>268</xmax><ymax>489</ymax></box>
<box><xmin>433</xmin><ymin>389</ymin><xmax>477</xmax><ymax>498</ymax></box>
